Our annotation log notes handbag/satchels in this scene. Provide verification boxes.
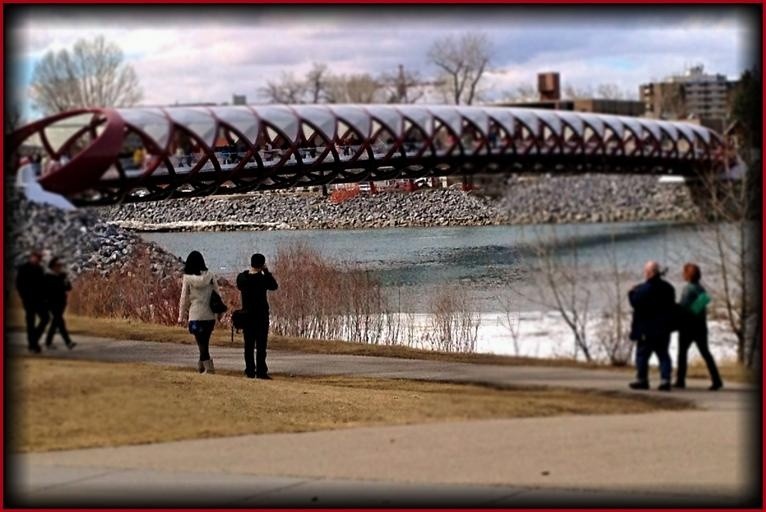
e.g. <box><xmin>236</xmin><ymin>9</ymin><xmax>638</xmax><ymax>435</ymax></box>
<box><xmin>232</xmin><ymin>310</ymin><xmax>242</xmax><ymax>329</ymax></box>
<box><xmin>210</xmin><ymin>278</ymin><xmax>227</xmax><ymax>313</ymax></box>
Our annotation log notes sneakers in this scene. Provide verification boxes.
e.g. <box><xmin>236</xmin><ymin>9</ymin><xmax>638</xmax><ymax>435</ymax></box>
<box><xmin>709</xmin><ymin>383</ymin><xmax>722</xmax><ymax>390</ymax></box>
<box><xmin>629</xmin><ymin>381</ymin><xmax>685</xmax><ymax>391</ymax></box>
<box><xmin>257</xmin><ymin>374</ymin><xmax>273</xmax><ymax>379</ymax></box>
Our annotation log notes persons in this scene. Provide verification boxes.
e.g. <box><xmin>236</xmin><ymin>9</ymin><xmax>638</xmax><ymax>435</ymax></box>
<box><xmin>13</xmin><ymin>252</ymin><xmax>48</xmax><ymax>357</ymax></box>
<box><xmin>626</xmin><ymin>261</ymin><xmax>676</xmax><ymax>391</ymax></box>
<box><xmin>42</xmin><ymin>255</ymin><xmax>78</xmax><ymax>351</ymax></box>
<box><xmin>176</xmin><ymin>251</ymin><xmax>223</xmax><ymax>375</ymax></box>
<box><xmin>236</xmin><ymin>253</ymin><xmax>278</xmax><ymax>380</ymax></box>
<box><xmin>126</xmin><ymin>127</ymin><xmax>501</xmax><ymax>170</ymax></box>
<box><xmin>673</xmin><ymin>261</ymin><xmax>723</xmax><ymax>390</ymax></box>
<box><xmin>13</xmin><ymin>145</ymin><xmax>74</xmax><ymax>194</ymax></box>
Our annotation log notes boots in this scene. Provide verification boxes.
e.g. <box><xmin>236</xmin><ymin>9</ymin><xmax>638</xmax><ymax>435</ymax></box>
<box><xmin>198</xmin><ymin>359</ymin><xmax>216</xmax><ymax>374</ymax></box>
